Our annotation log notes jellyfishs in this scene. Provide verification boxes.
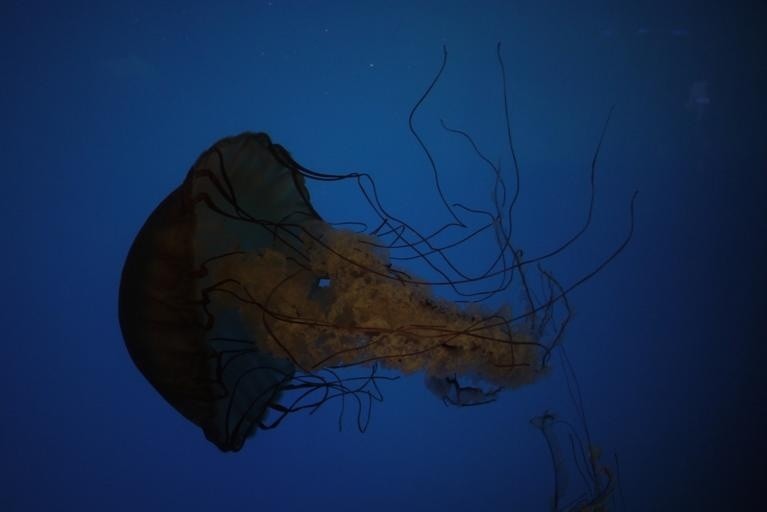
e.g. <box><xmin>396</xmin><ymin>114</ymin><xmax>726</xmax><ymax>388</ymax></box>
<box><xmin>116</xmin><ymin>40</ymin><xmax>640</xmax><ymax>452</ymax></box>
